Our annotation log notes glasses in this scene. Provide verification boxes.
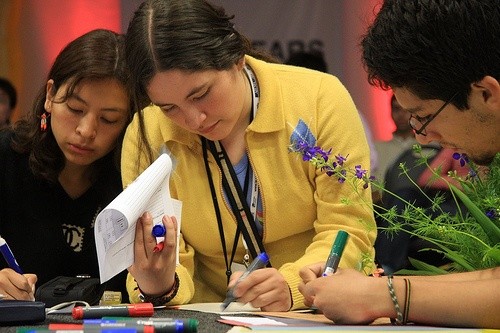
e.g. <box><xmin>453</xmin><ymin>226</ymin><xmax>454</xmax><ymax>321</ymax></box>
<box><xmin>408</xmin><ymin>91</ymin><xmax>462</xmax><ymax>136</ymax></box>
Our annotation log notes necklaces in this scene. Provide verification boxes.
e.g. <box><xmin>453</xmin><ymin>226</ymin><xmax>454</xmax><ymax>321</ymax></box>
<box><xmin>192</xmin><ymin>65</ymin><xmax>276</xmax><ymax>302</ymax></box>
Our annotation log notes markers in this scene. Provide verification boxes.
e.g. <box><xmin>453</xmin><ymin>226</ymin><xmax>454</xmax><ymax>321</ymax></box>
<box><xmin>73</xmin><ymin>302</ymin><xmax>153</xmax><ymax>320</ymax></box>
<box><xmin>152</xmin><ymin>222</ymin><xmax>166</xmax><ymax>237</ymax></box>
<box><xmin>153</xmin><ymin>242</ymin><xmax>164</xmax><ymax>253</ymax></box>
<box><xmin>16</xmin><ymin>317</ymin><xmax>198</xmax><ymax>333</ymax></box>
<box><xmin>312</xmin><ymin>230</ymin><xmax>350</xmax><ymax>315</ymax></box>
<box><xmin>0</xmin><ymin>236</ymin><xmax>35</xmax><ymax>302</ymax></box>
<box><xmin>221</xmin><ymin>252</ymin><xmax>270</xmax><ymax>313</ymax></box>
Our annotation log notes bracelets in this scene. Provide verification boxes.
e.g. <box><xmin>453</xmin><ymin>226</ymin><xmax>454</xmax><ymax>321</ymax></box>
<box><xmin>132</xmin><ymin>274</ymin><xmax>181</xmax><ymax>304</ymax></box>
<box><xmin>386</xmin><ymin>276</ymin><xmax>412</xmax><ymax>325</ymax></box>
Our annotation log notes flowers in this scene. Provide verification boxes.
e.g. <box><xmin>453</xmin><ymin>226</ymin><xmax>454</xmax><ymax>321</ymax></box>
<box><xmin>295</xmin><ymin>141</ymin><xmax>500</xmax><ymax>274</ymax></box>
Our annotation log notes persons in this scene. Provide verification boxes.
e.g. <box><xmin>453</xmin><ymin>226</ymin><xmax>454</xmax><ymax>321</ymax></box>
<box><xmin>298</xmin><ymin>0</ymin><xmax>500</xmax><ymax>329</ymax></box>
<box><xmin>0</xmin><ymin>29</ymin><xmax>150</xmax><ymax>301</ymax></box>
<box><xmin>379</xmin><ymin>96</ymin><xmax>487</xmax><ymax>196</ymax></box>
<box><xmin>0</xmin><ymin>79</ymin><xmax>18</xmax><ymax>130</ymax></box>
<box><xmin>357</xmin><ymin>110</ymin><xmax>378</xmax><ymax>203</ymax></box>
<box><xmin>119</xmin><ymin>0</ymin><xmax>377</xmax><ymax>311</ymax></box>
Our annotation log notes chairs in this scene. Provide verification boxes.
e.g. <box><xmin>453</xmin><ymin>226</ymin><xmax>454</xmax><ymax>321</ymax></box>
<box><xmin>371</xmin><ymin>144</ymin><xmax>474</xmax><ymax>270</ymax></box>
